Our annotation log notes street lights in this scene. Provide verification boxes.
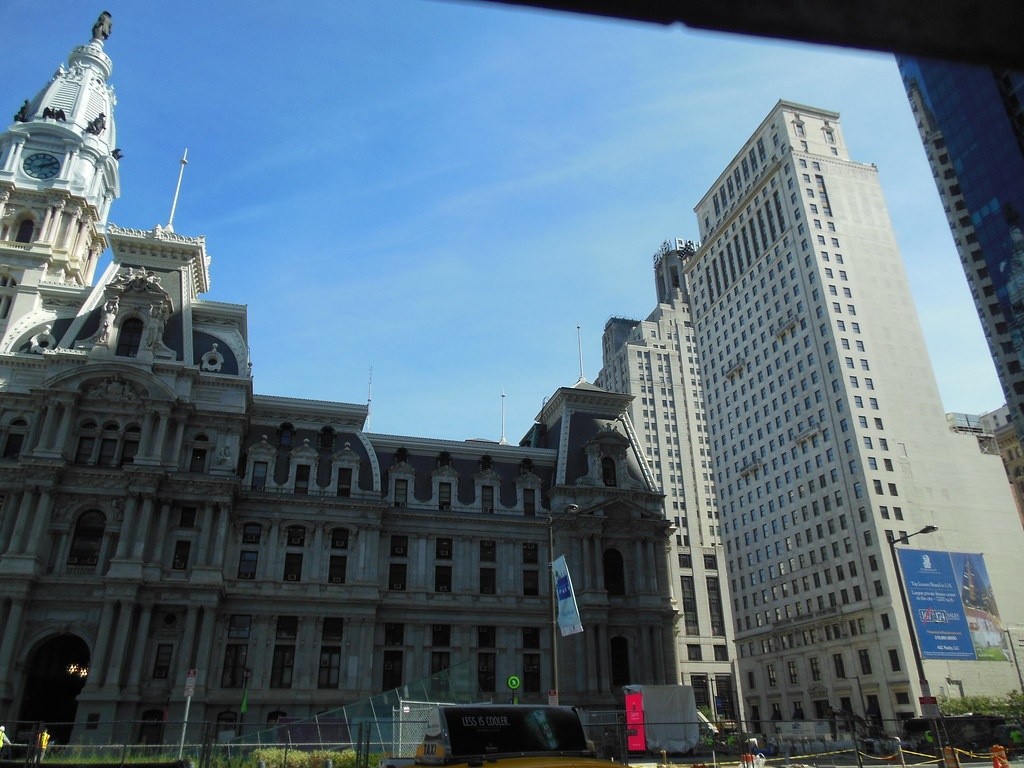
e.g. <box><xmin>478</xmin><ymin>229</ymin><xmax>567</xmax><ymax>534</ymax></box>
<box><xmin>887</xmin><ymin>525</ymin><xmax>949</xmax><ymax>768</ymax></box>
<box><xmin>547</xmin><ymin>502</ymin><xmax>579</xmax><ymax>704</ymax></box>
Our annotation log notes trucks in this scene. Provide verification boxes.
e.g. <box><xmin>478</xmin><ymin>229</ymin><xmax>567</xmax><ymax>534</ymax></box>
<box><xmin>381</xmin><ymin>702</ymin><xmax>636</xmax><ymax>768</ymax></box>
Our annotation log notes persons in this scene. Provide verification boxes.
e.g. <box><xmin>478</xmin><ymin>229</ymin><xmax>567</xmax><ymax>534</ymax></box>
<box><xmin>916</xmin><ymin>728</ymin><xmax>937</xmax><ymax>757</ymax></box>
<box><xmin>92</xmin><ymin>9</ymin><xmax>112</xmax><ymax>41</ymax></box>
<box><xmin>592</xmin><ymin>452</ymin><xmax>603</xmax><ymax>481</ymax></box>
<box><xmin>87</xmin><ymin>112</ymin><xmax>106</xmax><ymax>134</ymax></box>
<box><xmin>745</xmin><ymin>738</ymin><xmax>798</xmax><ymax>764</ymax></box>
<box><xmin>105</xmin><ymin>266</ymin><xmax>169</xmax><ymax>295</ymax></box>
<box><xmin>143</xmin><ymin>305</ymin><xmax>161</xmax><ymax>348</ymax></box>
<box><xmin>599</xmin><ymin>422</ymin><xmax>623</xmax><ymax>435</ymax></box>
<box><xmin>0</xmin><ymin>726</ymin><xmax>11</xmax><ymax>752</ymax></box>
<box><xmin>619</xmin><ymin>460</ymin><xmax>627</xmax><ymax>483</ymax></box>
<box><xmin>216</xmin><ymin>443</ymin><xmax>232</xmax><ymax>466</ymax></box>
<box><xmin>33</xmin><ymin>727</ymin><xmax>50</xmax><ymax>764</ymax></box>
<box><xmin>91</xmin><ymin>379</ymin><xmax>136</xmax><ymax>400</ymax></box>
<box><xmin>98</xmin><ymin>301</ymin><xmax>118</xmax><ymax>343</ymax></box>
<box><xmin>17</xmin><ymin>99</ymin><xmax>30</xmax><ymax>120</ymax></box>
